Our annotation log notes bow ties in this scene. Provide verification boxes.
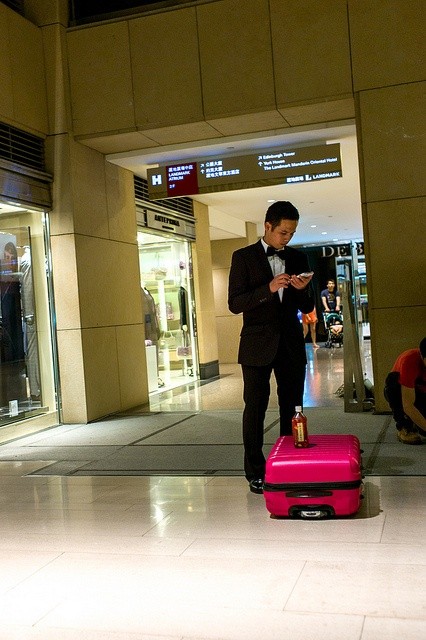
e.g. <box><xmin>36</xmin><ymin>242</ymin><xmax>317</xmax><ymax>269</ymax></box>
<box><xmin>266</xmin><ymin>247</ymin><xmax>285</xmax><ymax>260</ymax></box>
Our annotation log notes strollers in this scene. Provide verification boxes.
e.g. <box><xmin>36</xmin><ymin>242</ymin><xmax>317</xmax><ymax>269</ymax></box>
<box><xmin>324</xmin><ymin>310</ymin><xmax>344</xmax><ymax>349</ymax></box>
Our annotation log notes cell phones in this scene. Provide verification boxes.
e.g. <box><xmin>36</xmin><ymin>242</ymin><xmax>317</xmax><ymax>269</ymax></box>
<box><xmin>288</xmin><ymin>272</ymin><xmax>314</xmax><ymax>280</ymax></box>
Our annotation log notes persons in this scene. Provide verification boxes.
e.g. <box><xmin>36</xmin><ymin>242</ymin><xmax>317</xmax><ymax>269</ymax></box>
<box><xmin>302</xmin><ymin>306</ymin><xmax>320</xmax><ymax>348</ymax></box>
<box><xmin>0</xmin><ymin>241</ymin><xmax>22</xmax><ymax>360</ymax></box>
<box><xmin>227</xmin><ymin>201</ymin><xmax>315</xmax><ymax>496</ymax></box>
<box><xmin>179</xmin><ymin>276</ymin><xmax>197</xmax><ymax>376</ymax></box>
<box><xmin>136</xmin><ymin>279</ymin><xmax>165</xmax><ymax>388</ymax></box>
<box><xmin>330</xmin><ymin>321</ymin><xmax>343</xmax><ymax>338</ymax></box>
<box><xmin>384</xmin><ymin>338</ymin><xmax>425</xmax><ymax>445</ymax></box>
<box><xmin>320</xmin><ymin>279</ymin><xmax>341</xmax><ymax>347</ymax></box>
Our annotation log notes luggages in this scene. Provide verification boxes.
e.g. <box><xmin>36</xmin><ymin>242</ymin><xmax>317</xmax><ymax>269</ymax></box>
<box><xmin>263</xmin><ymin>434</ymin><xmax>365</xmax><ymax>520</ymax></box>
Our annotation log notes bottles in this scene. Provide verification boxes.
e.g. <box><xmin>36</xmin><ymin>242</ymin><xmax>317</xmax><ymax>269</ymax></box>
<box><xmin>291</xmin><ymin>405</ymin><xmax>310</xmax><ymax>448</ymax></box>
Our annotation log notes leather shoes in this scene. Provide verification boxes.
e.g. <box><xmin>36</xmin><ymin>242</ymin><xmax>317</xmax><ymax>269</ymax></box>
<box><xmin>250</xmin><ymin>479</ymin><xmax>263</xmax><ymax>493</ymax></box>
<box><xmin>397</xmin><ymin>427</ymin><xmax>425</xmax><ymax>444</ymax></box>
